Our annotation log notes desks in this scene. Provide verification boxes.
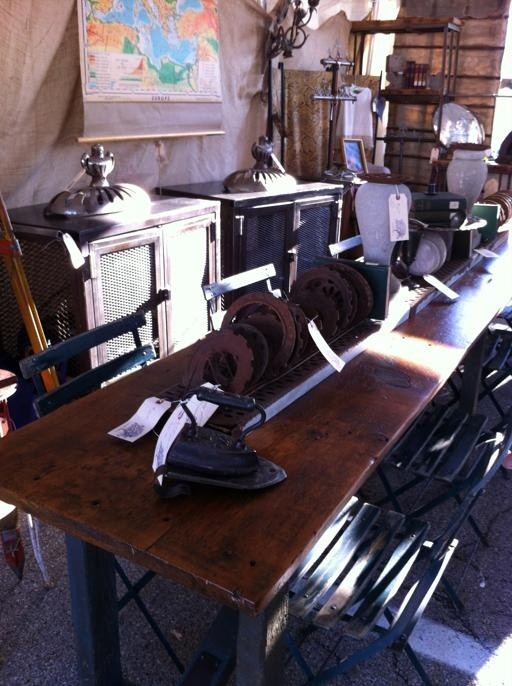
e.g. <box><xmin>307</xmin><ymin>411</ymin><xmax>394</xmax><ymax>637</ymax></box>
<box><xmin>2</xmin><ymin>232</ymin><xmax>511</xmax><ymax>685</ymax></box>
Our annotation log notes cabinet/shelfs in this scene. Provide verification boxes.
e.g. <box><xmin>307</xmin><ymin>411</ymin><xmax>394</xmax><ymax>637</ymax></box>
<box><xmin>157</xmin><ymin>172</ymin><xmax>346</xmax><ymax>306</ymax></box>
<box><xmin>344</xmin><ymin>15</ymin><xmax>464</xmax><ymax>187</ymax></box>
<box><xmin>2</xmin><ymin>197</ymin><xmax>222</xmax><ymax>386</ymax></box>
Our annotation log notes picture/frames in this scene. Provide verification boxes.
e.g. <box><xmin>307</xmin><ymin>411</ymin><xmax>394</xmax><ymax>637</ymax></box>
<box><xmin>339</xmin><ymin>136</ymin><xmax>369</xmax><ymax>175</ymax></box>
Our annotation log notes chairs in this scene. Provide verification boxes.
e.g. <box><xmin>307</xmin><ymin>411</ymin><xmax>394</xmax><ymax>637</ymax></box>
<box><xmin>201</xmin><ymin>262</ymin><xmax>284</xmax><ymax>330</ymax></box>
